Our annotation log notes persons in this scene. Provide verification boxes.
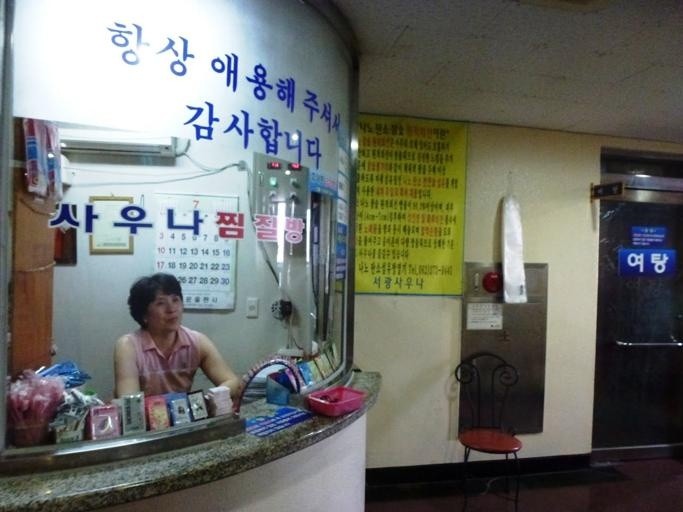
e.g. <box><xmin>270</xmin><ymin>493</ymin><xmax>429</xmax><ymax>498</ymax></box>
<box><xmin>114</xmin><ymin>273</ymin><xmax>240</xmax><ymax>403</ymax></box>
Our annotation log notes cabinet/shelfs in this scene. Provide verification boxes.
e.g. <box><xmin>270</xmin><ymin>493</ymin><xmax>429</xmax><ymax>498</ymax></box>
<box><xmin>7</xmin><ymin>115</ymin><xmax>57</xmax><ymax>383</ymax></box>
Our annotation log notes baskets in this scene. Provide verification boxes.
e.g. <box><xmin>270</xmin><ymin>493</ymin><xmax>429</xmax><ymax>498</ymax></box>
<box><xmin>307</xmin><ymin>386</ymin><xmax>366</xmax><ymax>418</ymax></box>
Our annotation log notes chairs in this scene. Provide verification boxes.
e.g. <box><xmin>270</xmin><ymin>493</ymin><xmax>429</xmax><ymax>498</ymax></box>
<box><xmin>455</xmin><ymin>350</ymin><xmax>523</xmax><ymax>512</ymax></box>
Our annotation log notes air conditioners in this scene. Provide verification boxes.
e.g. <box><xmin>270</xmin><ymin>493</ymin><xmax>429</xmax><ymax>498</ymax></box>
<box><xmin>57</xmin><ymin>122</ymin><xmax>177</xmax><ymax>159</ymax></box>
